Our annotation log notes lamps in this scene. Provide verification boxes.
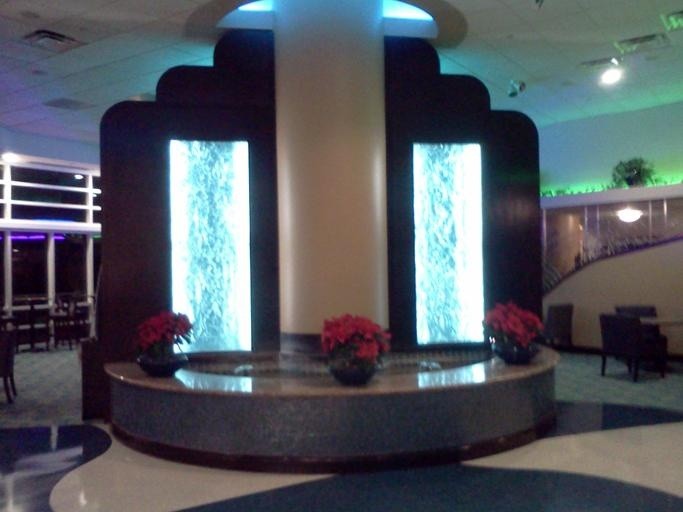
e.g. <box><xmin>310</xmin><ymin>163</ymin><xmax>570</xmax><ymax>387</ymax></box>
<box><xmin>506</xmin><ymin>80</ymin><xmax>526</xmax><ymax>97</ymax></box>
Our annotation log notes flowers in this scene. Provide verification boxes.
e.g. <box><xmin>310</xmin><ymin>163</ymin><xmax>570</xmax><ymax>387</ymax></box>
<box><xmin>319</xmin><ymin>308</ymin><xmax>393</xmax><ymax>370</ymax></box>
<box><xmin>126</xmin><ymin>310</ymin><xmax>190</xmax><ymax>357</ymax></box>
<box><xmin>482</xmin><ymin>296</ymin><xmax>546</xmax><ymax>349</ymax></box>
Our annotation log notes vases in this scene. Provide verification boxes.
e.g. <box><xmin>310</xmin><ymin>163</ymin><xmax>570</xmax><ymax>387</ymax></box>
<box><xmin>491</xmin><ymin>347</ymin><xmax>539</xmax><ymax>365</ymax></box>
<box><xmin>328</xmin><ymin>368</ymin><xmax>376</xmax><ymax>386</ymax></box>
<box><xmin>135</xmin><ymin>354</ymin><xmax>189</xmax><ymax>378</ymax></box>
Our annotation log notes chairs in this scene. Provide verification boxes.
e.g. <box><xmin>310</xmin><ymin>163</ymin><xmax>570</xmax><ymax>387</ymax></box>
<box><xmin>10</xmin><ymin>296</ymin><xmax>95</xmax><ymax>352</ymax></box>
<box><xmin>0</xmin><ymin>315</ymin><xmax>16</xmax><ymax>404</ymax></box>
<box><xmin>542</xmin><ymin>303</ymin><xmax>681</xmax><ymax>381</ymax></box>
